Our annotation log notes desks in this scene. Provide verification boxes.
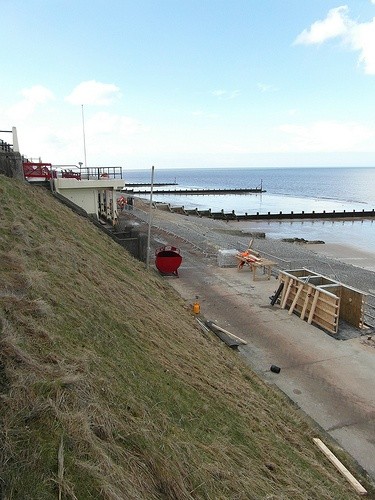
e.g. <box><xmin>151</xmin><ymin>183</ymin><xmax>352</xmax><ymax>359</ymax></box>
<box><xmin>237</xmin><ymin>255</ymin><xmax>277</xmax><ymax>280</ymax></box>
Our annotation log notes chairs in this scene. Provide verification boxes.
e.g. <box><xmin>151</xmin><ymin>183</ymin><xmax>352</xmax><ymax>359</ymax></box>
<box><xmin>60</xmin><ymin>170</ymin><xmax>81</xmax><ymax>181</ymax></box>
